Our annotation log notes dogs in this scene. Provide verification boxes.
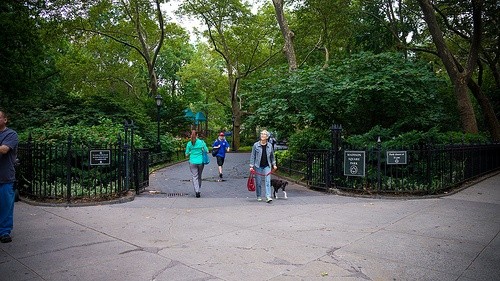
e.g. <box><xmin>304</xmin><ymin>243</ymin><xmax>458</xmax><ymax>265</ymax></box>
<box><xmin>271</xmin><ymin>179</ymin><xmax>288</xmax><ymax>198</ymax></box>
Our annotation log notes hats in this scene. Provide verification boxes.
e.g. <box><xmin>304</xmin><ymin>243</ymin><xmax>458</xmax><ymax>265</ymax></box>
<box><xmin>219</xmin><ymin>133</ymin><xmax>225</xmax><ymax>136</ymax></box>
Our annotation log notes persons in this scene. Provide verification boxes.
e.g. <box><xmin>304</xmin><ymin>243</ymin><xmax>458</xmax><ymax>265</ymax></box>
<box><xmin>212</xmin><ymin>133</ymin><xmax>230</xmax><ymax>178</ymax></box>
<box><xmin>250</xmin><ymin>130</ymin><xmax>277</xmax><ymax>203</ymax></box>
<box><xmin>222</xmin><ymin>127</ymin><xmax>227</xmax><ymax>132</ymax></box>
<box><xmin>0</xmin><ymin>108</ymin><xmax>19</xmax><ymax>243</ymax></box>
<box><xmin>185</xmin><ymin>129</ymin><xmax>208</xmax><ymax>197</ymax></box>
<box><xmin>269</xmin><ymin>134</ymin><xmax>276</xmax><ymax>150</ymax></box>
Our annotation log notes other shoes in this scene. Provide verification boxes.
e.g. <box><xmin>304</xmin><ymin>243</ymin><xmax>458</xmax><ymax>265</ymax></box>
<box><xmin>2</xmin><ymin>234</ymin><xmax>12</xmax><ymax>244</ymax></box>
<box><xmin>219</xmin><ymin>173</ymin><xmax>223</xmax><ymax>178</ymax></box>
<box><xmin>256</xmin><ymin>197</ymin><xmax>263</xmax><ymax>201</ymax></box>
<box><xmin>196</xmin><ymin>193</ymin><xmax>200</xmax><ymax>198</ymax></box>
<box><xmin>265</xmin><ymin>196</ymin><xmax>273</xmax><ymax>203</ymax></box>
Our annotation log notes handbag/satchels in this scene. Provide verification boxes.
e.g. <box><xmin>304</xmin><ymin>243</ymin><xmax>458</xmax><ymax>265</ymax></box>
<box><xmin>247</xmin><ymin>170</ymin><xmax>256</xmax><ymax>191</ymax></box>
<box><xmin>212</xmin><ymin>148</ymin><xmax>219</xmax><ymax>157</ymax></box>
<box><xmin>202</xmin><ymin>151</ymin><xmax>210</xmax><ymax>164</ymax></box>
